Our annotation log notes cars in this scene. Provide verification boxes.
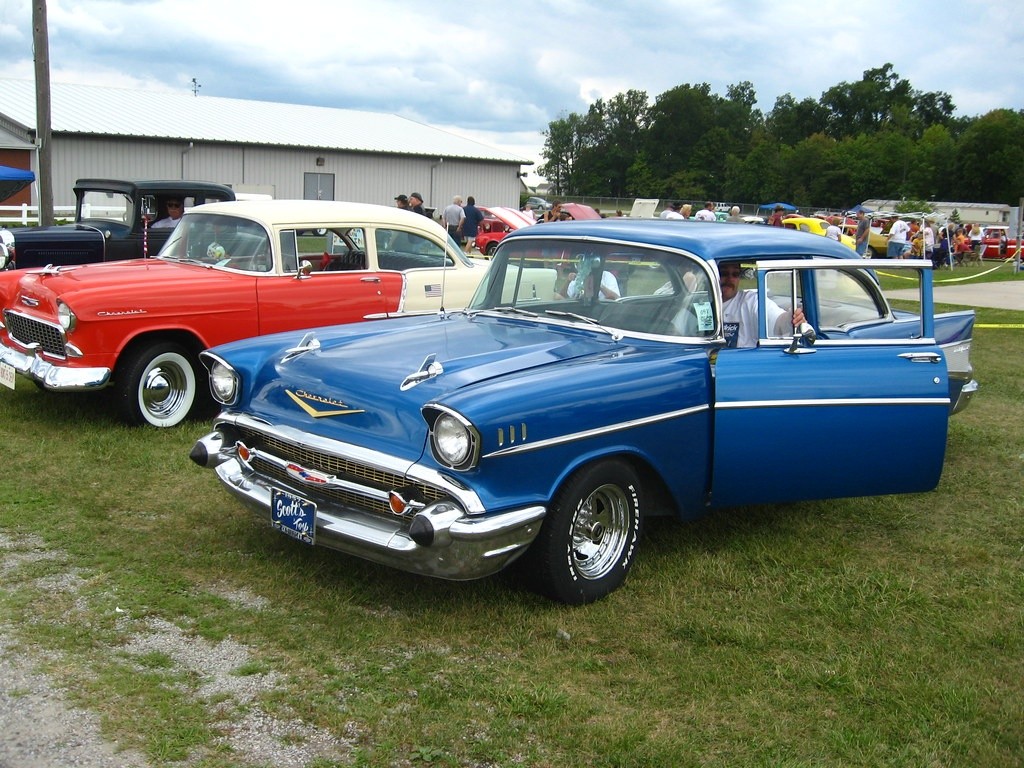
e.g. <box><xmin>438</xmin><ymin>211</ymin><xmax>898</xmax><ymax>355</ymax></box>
<box><xmin>188</xmin><ymin>216</ymin><xmax>979</xmax><ymax>611</ymax></box>
<box><xmin>0</xmin><ymin>177</ymin><xmax>238</xmax><ymax>273</ymax></box>
<box><xmin>463</xmin><ymin>201</ymin><xmax>1024</xmax><ymax>260</ymax></box>
<box><xmin>0</xmin><ymin>198</ymin><xmax>565</xmax><ymax>431</ymax></box>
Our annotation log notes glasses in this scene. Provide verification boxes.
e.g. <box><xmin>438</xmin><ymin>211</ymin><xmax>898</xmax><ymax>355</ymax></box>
<box><xmin>720</xmin><ymin>271</ymin><xmax>739</xmax><ymax>277</ymax></box>
<box><xmin>167</xmin><ymin>203</ymin><xmax>181</xmax><ymax>208</ymax></box>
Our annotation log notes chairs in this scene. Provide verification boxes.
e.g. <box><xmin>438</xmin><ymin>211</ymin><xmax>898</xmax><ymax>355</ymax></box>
<box><xmin>931</xmin><ymin>245</ymin><xmax>988</xmax><ymax>270</ymax></box>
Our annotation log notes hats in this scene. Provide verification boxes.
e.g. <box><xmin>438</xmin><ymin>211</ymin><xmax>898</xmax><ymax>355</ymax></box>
<box><xmin>411</xmin><ymin>193</ymin><xmax>423</xmax><ymax>203</ymax></box>
<box><xmin>671</xmin><ymin>203</ymin><xmax>682</xmax><ymax>208</ymax></box>
<box><xmin>774</xmin><ymin>206</ymin><xmax>786</xmax><ymax>211</ymax></box>
<box><xmin>395</xmin><ymin>195</ymin><xmax>407</xmax><ymax>201</ymax></box>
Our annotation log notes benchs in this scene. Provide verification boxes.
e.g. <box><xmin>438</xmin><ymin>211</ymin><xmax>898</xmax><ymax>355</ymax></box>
<box><xmin>589</xmin><ymin>298</ymin><xmax>663</xmax><ymax>332</ymax></box>
<box><xmin>323</xmin><ymin>249</ymin><xmax>452</xmax><ymax>273</ymax></box>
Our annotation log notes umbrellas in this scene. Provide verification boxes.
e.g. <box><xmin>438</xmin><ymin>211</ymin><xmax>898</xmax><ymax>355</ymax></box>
<box><xmin>847</xmin><ymin>205</ymin><xmax>872</xmax><ymax>213</ymax></box>
<box><xmin>0</xmin><ymin>166</ymin><xmax>36</xmax><ymax>204</ymax></box>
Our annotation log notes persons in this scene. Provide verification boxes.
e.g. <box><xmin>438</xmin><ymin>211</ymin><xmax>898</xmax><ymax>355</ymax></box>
<box><xmin>150</xmin><ymin>193</ymin><xmax>195</xmax><ymax>229</ymax></box>
<box><xmin>1000</xmin><ymin>230</ymin><xmax>1008</xmax><ymax>260</ymax></box>
<box><xmin>660</xmin><ymin>201</ymin><xmax>684</xmax><ymax>220</ymax></box>
<box><xmin>871</xmin><ymin>215</ymin><xmax>990</xmax><ymax>270</ymax></box>
<box><xmin>594</xmin><ymin>208</ymin><xmax>622</xmax><ymax>218</ymax></box>
<box><xmin>463</xmin><ymin>196</ymin><xmax>484</xmax><ymax>255</ymax></box>
<box><xmin>671</xmin><ymin>261</ymin><xmax>809</xmax><ymax>349</ymax></box>
<box><xmin>441</xmin><ymin>196</ymin><xmax>466</xmax><ymax>248</ymax></box>
<box><xmin>536</xmin><ymin>200</ymin><xmax>570</xmax><ymax>224</ymax></box>
<box><xmin>825</xmin><ymin>218</ymin><xmax>842</xmax><ymax>242</ymax></box>
<box><xmin>768</xmin><ymin>206</ymin><xmax>786</xmax><ymax>227</ymax></box>
<box><xmin>694</xmin><ymin>202</ymin><xmax>717</xmax><ymax>222</ymax></box>
<box><xmin>679</xmin><ymin>204</ymin><xmax>695</xmax><ymax>221</ymax></box>
<box><xmin>555</xmin><ymin>270</ymin><xmax>620</xmax><ymax>300</ymax></box>
<box><xmin>726</xmin><ymin>206</ymin><xmax>745</xmax><ymax>223</ymax></box>
<box><xmin>854</xmin><ymin>210</ymin><xmax>870</xmax><ymax>257</ymax></box>
<box><xmin>396</xmin><ymin>192</ymin><xmax>426</xmax><ymax>253</ymax></box>
<box><xmin>523</xmin><ymin>204</ymin><xmax>536</xmax><ymax>220</ymax></box>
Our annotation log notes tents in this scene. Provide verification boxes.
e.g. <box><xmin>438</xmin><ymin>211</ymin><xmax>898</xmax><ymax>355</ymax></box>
<box><xmin>756</xmin><ymin>203</ymin><xmax>797</xmax><ymax>217</ymax></box>
<box><xmin>842</xmin><ymin>211</ymin><xmax>954</xmax><ymax>272</ymax></box>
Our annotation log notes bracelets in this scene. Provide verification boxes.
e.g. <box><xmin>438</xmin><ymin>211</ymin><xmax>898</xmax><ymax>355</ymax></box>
<box><xmin>861</xmin><ymin>236</ymin><xmax>864</xmax><ymax>241</ymax></box>
